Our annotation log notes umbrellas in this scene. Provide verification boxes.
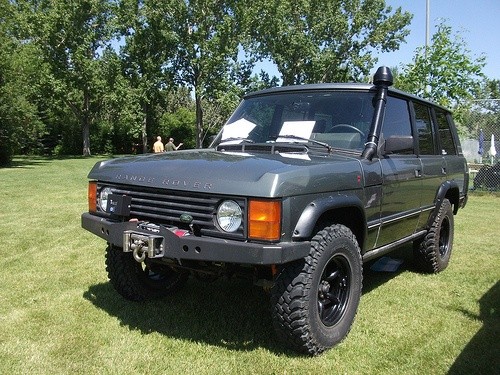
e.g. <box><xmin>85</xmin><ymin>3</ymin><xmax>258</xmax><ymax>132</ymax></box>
<box><xmin>476</xmin><ymin>128</ymin><xmax>485</xmax><ymax>164</ymax></box>
<box><xmin>487</xmin><ymin>134</ymin><xmax>497</xmax><ymax>166</ymax></box>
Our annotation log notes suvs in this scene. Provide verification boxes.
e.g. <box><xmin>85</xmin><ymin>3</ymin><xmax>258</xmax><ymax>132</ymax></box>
<box><xmin>80</xmin><ymin>64</ymin><xmax>470</xmax><ymax>356</ymax></box>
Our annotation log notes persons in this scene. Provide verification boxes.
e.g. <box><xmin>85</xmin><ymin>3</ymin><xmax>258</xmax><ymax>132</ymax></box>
<box><xmin>165</xmin><ymin>137</ymin><xmax>184</xmax><ymax>152</ymax></box>
<box><xmin>152</xmin><ymin>136</ymin><xmax>164</xmax><ymax>154</ymax></box>
